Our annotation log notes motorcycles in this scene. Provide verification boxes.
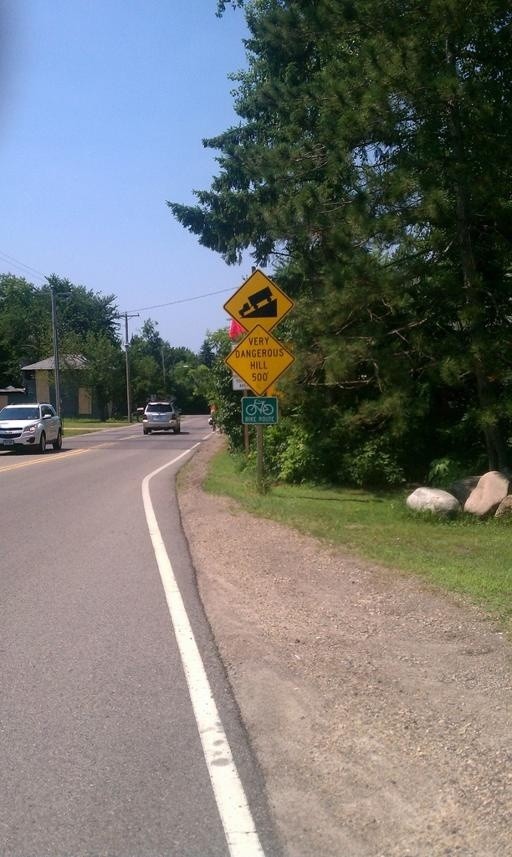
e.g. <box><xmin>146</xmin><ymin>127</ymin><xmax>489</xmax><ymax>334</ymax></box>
<box><xmin>209</xmin><ymin>413</ymin><xmax>217</xmax><ymax>431</ymax></box>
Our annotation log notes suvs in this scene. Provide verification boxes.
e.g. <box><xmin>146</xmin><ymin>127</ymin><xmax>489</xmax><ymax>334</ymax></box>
<box><xmin>142</xmin><ymin>401</ymin><xmax>180</xmax><ymax>433</ymax></box>
<box><xmin>0</xmin><ymin>400</ymin><xmax>63</xmax><ymax>452</ymax></box>
